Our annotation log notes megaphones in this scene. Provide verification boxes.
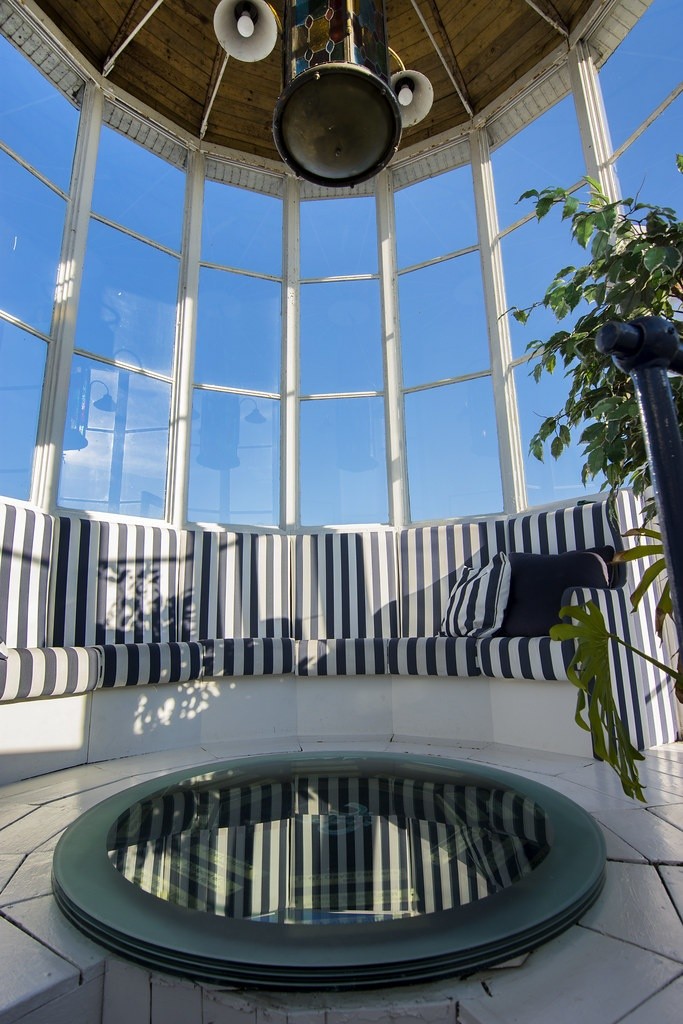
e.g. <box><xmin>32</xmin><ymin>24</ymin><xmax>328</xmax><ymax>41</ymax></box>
<box><xmin>391</xmin><ymin>70</ymin><xmax>434</xmax><ymax>129</ymax></box>
<box><xmin>213</xmin><ymin>0</ymin><xmax>278</xmax><ymax>62</ymax></box>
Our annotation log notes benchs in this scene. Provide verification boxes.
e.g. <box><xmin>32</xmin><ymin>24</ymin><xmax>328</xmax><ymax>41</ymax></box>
<box><xmin>0</xmin><ymin>488</ymin><xmax>677</xmax><ymax>784</ymax></box>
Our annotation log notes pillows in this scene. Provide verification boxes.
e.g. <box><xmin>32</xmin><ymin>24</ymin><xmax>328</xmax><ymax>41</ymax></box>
<box><xmin>436</xmin><ymin>552</ymin><xmax>511</xmax><ymax>637</ymax></box>
<box><xmin>492</xmin><ymin>545</ymin><xmax>615</xmax><ymax>637</ymax></box>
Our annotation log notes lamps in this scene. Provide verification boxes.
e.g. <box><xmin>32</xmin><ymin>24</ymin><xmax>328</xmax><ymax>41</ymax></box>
<box><xmin>388</xmin><ymin>47</ymin><xmax>434</xmax><ymax>129</ymax></box>
<box><xmin>213</xmin><ymin>0</ymin><xmax>282</xmax><ymax>62</ymax></box>
